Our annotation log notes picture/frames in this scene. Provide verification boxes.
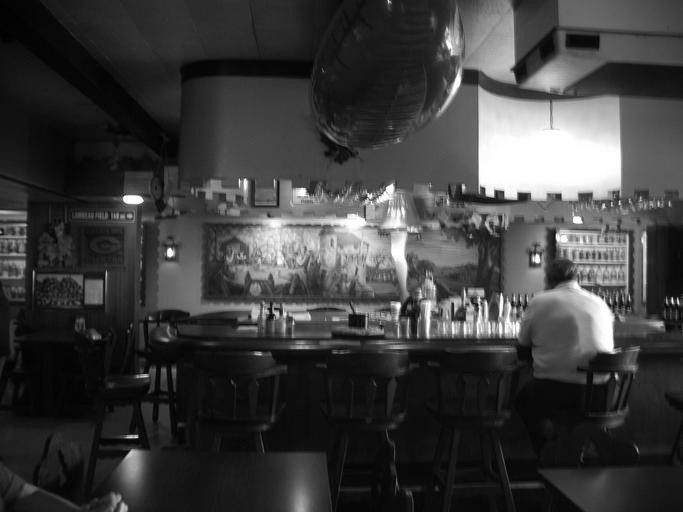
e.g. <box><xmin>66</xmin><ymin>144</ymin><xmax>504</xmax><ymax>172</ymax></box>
<box><xmin>79</xmin><ymin>225</ymin><xmax>127</xmax><ymax>269</ymax></box>
<box><xmin>31</xmin><ymin>270</ymin><xmax>84</xmax><ymax>314</ymax></box>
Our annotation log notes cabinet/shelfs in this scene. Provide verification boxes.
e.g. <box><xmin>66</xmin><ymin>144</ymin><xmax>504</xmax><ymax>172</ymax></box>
<box><xmin>553</xmin><ymin>229</ymin><xmax>634</xmax><ymax>311</ymax></box>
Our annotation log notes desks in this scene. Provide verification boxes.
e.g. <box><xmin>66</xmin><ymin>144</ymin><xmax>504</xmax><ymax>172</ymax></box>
<box><xmin>537</xmin><ymin>466</ymin><xmax>682</xmax><ymax>512</ymax></box>
<box><xmin>91</xmin><ymin>449</ymin><xmax>332</xmax><ymax>512</ymax></box>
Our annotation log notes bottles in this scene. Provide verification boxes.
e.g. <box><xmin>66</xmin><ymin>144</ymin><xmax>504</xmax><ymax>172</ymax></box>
<box><xmin>257</xmin><ymin>299</ymin><xmax>296</xmax><ymax>334</ymax></box>
<box><xmin>505</xmin><ymin>293</ymin><xmax>528</xmax><ymax>316</ymax></box>
<box><xmin>577</xmin><ymin>261</ymin><xmax>631</xmax><ymax>312</ymax></box>
<box><xmin>663</xmin><ymin>296</ymin><xmax>683</xmax><ymax>322</ymax></box>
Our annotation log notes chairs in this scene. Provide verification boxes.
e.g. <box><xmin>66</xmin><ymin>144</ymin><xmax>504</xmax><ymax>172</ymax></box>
<box><xmin>85</xmin><ymin>310</ymin><xmax>190</xmax><ymax>502</ymax></box>
<box><xmin>310</xmin><ymin>347</ymin><xmax>411</xmax><ymax>507</ymax></box>
<box><xmin>194</xmin><ymin>346</ymin><xmax>302</xmax><ymax>453</ymax></box>
<box><xmin>538</xmin><ymin>347</ymin><xmax>638</xmax><ymax>508</ymax></box>
<box><xmin>414</xmin><ymin>347</ymin><xmax>523</xmax><ymax>508</ymax></box>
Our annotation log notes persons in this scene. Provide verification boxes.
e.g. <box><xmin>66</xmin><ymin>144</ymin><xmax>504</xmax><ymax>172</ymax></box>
<box><xmin>0</xmin><ymin>464</ymin><xmax>129</xmax><ymax>512</ymax></box>
<box><xmin>514</xmin><ymin>257</ymin><xmax>615</xmax><ymax>460</ymax></box>
